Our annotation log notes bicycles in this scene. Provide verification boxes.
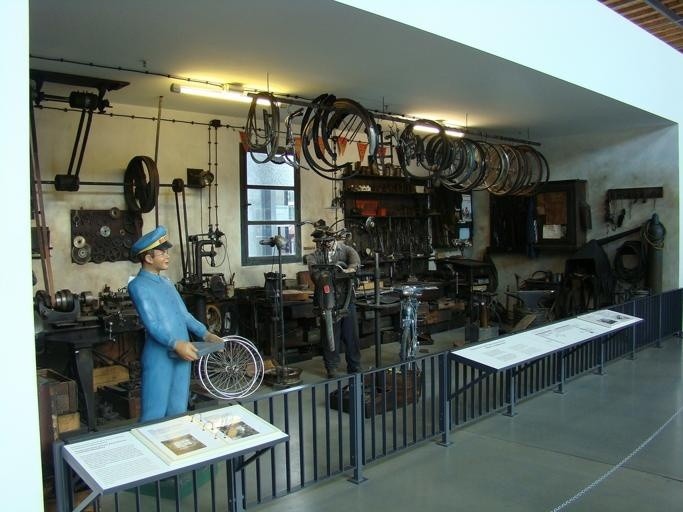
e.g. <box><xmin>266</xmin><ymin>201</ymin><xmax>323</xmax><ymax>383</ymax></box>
<box><xmin>297</xmin><ymin>220</ymin><xmax>364</xmax><ymax>353</ymax></box>
<box><xmin>394</xmin><ymin>285</ymin><xmax>422</xmax><ymax>375</ymax></box>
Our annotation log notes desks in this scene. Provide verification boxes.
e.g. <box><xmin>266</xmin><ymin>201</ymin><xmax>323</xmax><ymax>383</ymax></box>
<box><xmin>241</xmin><ymin>276</ymin><xmax>467</xmax><ymax>366</ymax></box>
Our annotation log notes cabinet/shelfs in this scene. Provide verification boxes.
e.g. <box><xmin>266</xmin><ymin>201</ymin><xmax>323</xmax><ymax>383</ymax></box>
<box><xmin>345</xmin><ymin>175</ymin><xmax>473</xmax><ymax>262</ymax></box>
<box><xmin>490</xmin><ymin>181</ymin><xmax>587</xmax><ymax>249</ymax></box>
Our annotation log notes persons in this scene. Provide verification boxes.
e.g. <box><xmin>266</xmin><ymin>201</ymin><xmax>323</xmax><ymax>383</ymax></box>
<box><xmin>124</xmin><ymin>226</ymin><xmax>224</xmax><ymax>420</ymax></box>
<box><xmin>304</xmin><ymin>228</ymin><xmax>360</xmax><ymax>385</ymax></box>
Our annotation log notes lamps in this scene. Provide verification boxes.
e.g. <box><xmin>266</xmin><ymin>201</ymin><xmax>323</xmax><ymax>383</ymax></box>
<box><xmin>171</xmin><ymin>84</ymin><xmax>281</xmax><ymax>107</ymax></box>
